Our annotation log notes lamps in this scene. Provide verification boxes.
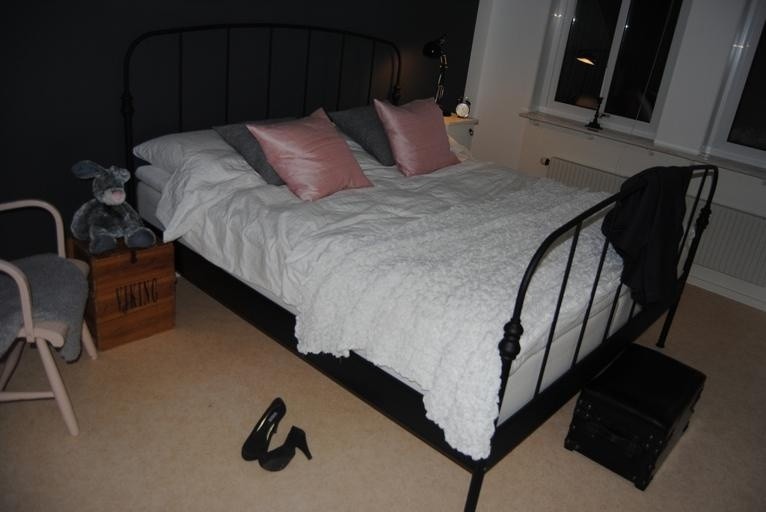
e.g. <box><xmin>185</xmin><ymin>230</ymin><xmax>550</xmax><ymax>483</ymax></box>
<box><xmin>421</xmin><ymin>29</ymin><xmax>452</xmax><ymax>119</ymax></box>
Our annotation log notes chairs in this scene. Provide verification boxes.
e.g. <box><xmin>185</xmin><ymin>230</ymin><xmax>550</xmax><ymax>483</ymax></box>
<box><xmin>0</xmin><ymin>197</ymin><xmax>99</xmax><ymax>439</ymax></box>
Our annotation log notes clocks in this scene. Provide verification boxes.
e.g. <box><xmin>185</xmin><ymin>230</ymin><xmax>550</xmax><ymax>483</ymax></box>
<box><xmin>452</xmin><ymin>95</ymin><xmax>473</xmax><ymax>120</ymax></box>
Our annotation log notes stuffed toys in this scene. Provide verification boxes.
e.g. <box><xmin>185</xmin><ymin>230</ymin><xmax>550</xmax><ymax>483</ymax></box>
<box><xmin>68</xmin><ymin>158</ymin><xmax>158</xmax><ymax>257</ymax></box>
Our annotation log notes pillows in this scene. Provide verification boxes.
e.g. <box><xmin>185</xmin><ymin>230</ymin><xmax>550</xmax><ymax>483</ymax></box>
<box><xmin>325</xmin><ymin>105</ymin><xmax>397</xmax><ymax>169</ymax></box>
<box><xmin>372</xmin><ymin>92</ymin><xmax>462</xmax><ymax>178</ymax></box>
<box><xmin>210</xmin><ymin>116</ymin><xmax>301</xmax><ymax>187</ymax></box>
<box><xmin>245</xmin><ymin>103</ymin><xmax>376</xmax><ymax>202</ymax></box>
<box><xmin>131</xmin><ymin>127</ymin><xmax>211</xmax><ymax>174</ymax></box>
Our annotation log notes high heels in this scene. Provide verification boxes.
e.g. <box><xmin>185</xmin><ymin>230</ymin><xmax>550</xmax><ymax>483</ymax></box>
<box><xmin>241</xmin><ymin>398</ymin><xmax>286</xmax><ymax>461</ymax></box>
<box><xmin>259</xmin><ymin>425</ymin><xmax>311</xmax><ymax>472</ymax></box>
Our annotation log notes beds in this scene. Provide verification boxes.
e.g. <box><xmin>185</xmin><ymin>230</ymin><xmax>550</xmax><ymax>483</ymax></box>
<box><xmin>117</xmin><ymin>19</ymin><xmax>720</xmax><ymax>510</ymax></box>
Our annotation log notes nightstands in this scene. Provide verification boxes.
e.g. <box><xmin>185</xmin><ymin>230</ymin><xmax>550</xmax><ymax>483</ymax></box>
<box><xmin>443</xmin><ymin>112</ymin><xmax>479</xmax><ymax>154</ymax></box>
<box><xmin>66</xmin><ymin>231</ymin><xmax>176</xmax><ymax>354</ymax></box>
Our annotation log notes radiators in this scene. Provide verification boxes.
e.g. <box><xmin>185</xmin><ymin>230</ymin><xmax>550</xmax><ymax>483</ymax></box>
<box><xmin>538</xmin><ymin>153</ymin><xmax>764</xmax><ymax>290</ymax></box>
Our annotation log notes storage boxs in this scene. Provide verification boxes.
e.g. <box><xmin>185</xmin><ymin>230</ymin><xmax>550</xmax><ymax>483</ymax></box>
<box><xmin>559</xmin><ymin>339</ymin><xmax>707</xmax><ymax>492</ymax></box>
